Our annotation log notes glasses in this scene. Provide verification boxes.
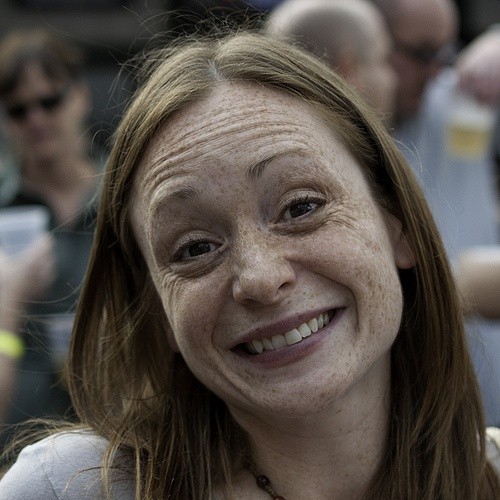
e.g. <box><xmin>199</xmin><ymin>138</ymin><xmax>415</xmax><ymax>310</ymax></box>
<box><xmin>393</xmin><ymin>40</ymin><xmax>443</xmax><ymax>65</ymax></box>
<box><xmin>7</xmin><ymin>88</ymin><xmax>73</xmax><ymax>125</ymax></box>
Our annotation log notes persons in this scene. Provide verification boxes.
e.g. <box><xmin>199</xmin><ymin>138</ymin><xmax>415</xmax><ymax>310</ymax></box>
<box><xmin>0</xmin><ymin>1</ymin><xmax>498</xmax><ymax>437</ymax></box>
<box><xmin>2</xmin><ymin>28</ymin><xmax>499</xmax><ymax>499</ymax></box>
